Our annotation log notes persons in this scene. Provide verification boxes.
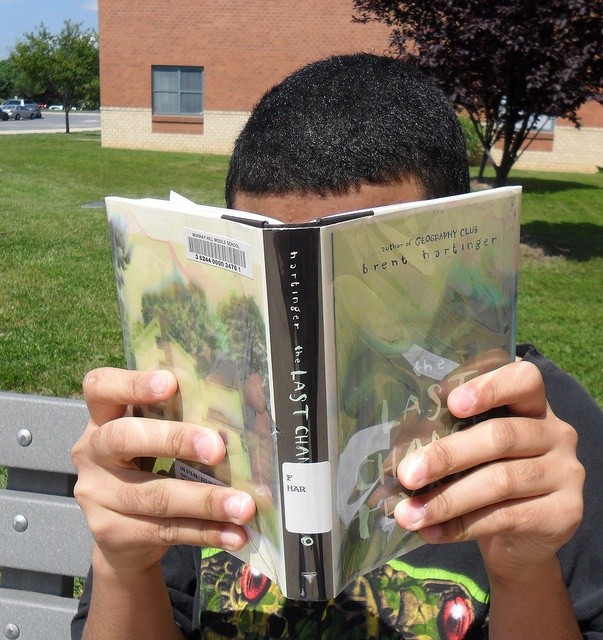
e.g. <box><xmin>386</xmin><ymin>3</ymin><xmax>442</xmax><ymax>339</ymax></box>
<box><xmin>63</xmin><ymin>50</ymin><xmax>603</xmax><ymax>640</ymax></box>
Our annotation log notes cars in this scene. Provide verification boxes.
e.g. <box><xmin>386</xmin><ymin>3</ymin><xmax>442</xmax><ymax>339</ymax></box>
<box><xmin>0</xmin><ymin>98</ymin><xmax>41</xmax><ymax>118</ymax></box>
<box><xmin>3</xmin><ymin>105</ymin><xmax>35</xmax><ymax>120</ymax></box>
<box><xmin>0</xmin><ymin>108</ymin><xmax>8</xmax><ymax>121</ymax></box>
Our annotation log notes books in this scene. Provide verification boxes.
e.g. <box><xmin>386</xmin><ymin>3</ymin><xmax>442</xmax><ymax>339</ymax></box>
<box><xmin>101</xmin><ymin>183</ymin><xmax>523</xmax><ymax>604</ymax></box>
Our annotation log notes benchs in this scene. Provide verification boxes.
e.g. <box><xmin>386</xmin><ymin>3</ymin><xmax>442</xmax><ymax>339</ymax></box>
<box><xmin>0</xmin><ymin>392</ymin><xmax>93</xmax><ymax>640</ymax></box>
